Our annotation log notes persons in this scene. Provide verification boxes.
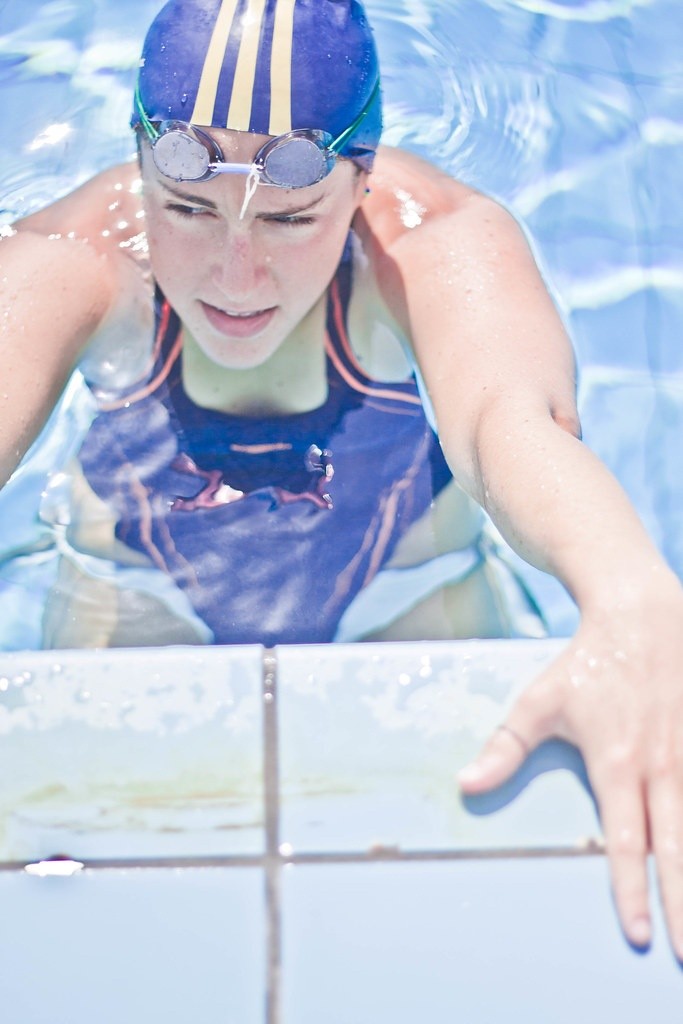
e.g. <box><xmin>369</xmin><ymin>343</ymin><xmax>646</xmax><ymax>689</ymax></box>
<box><xmin>0</xmin><ymin>0</ymin><xmax>682</xmax><ymax>975</ymax></box>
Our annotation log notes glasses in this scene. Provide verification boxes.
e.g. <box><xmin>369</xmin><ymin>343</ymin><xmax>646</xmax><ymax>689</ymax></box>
<box><xmin>152</xmin><ymin>120</ymin><xmax>336</xmax><ymax>189</ymax></box>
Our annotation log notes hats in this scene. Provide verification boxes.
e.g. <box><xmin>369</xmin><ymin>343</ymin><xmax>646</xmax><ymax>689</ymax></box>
<box><xmin>130</xmin><ymin>0</ymin><xmax>382</xmax><ymax>156</ymax></box>
